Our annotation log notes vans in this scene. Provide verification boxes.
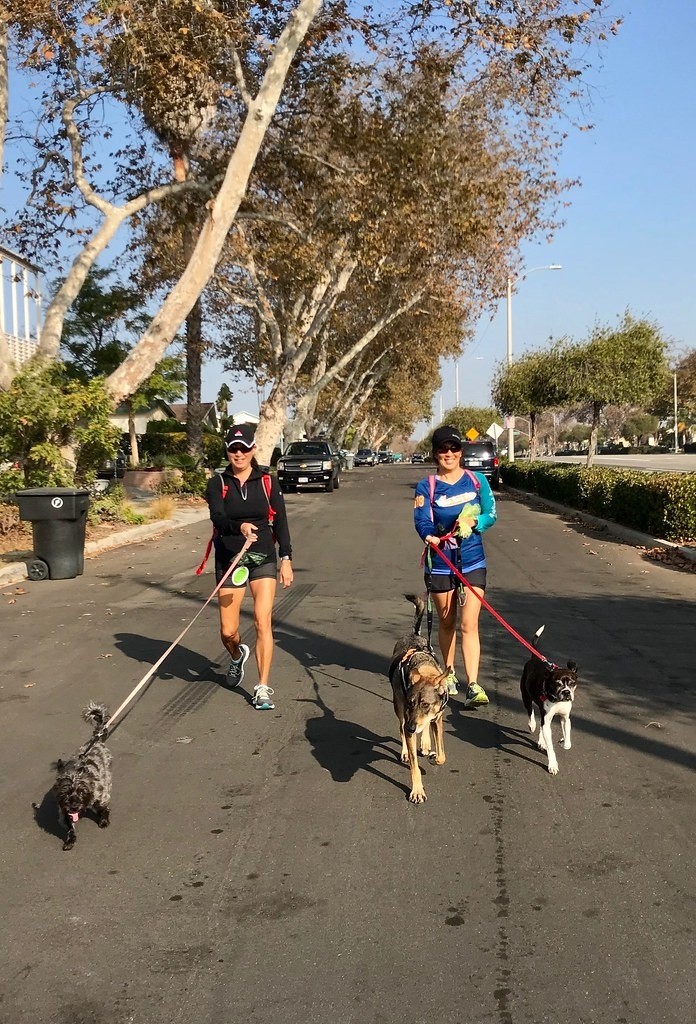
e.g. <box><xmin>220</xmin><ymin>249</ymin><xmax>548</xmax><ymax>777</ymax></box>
<box><xmin>409</xmin><ymin>452</ymin><xmax>425</xmax><ymax>464</ymax></box>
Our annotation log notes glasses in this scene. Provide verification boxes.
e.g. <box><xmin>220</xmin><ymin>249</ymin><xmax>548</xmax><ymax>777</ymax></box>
<box><xmin>436</xmin><ymin>443</ymin><xmax>461</xmax><ymax>453</ymax></box>
<box><xmin>227</xmin><ymin>446</ymin><xmax>252</xmax><ymax>453</ymax></box>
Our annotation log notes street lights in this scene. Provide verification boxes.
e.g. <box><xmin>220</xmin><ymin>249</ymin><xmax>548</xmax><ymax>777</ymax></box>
<box><xmin>455</xmin><ymin>353</ymin><xmax>483</xmax><ymax>409</ymax></box>
<box><xmin>507</xmin><ymin>263</ymin><xmax>562</xmax><ymax>374</ymax></box>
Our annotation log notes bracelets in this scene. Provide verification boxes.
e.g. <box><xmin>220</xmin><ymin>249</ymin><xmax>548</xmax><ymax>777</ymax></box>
<box><xmin>471</xmin><ymin>516</ymin><xmax>478</xmax><ymax>529</ymax></box>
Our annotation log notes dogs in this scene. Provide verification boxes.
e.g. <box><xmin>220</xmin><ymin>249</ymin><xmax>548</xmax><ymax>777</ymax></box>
<box><xmin>53</xmin><ymin>698</ymin><xmax>113</xmax><ymax>851</ymax></box>
<box><xmin>387</xmin><ymin>593</ymin><xmax>451</xmax><ymax>805</ymax></box>
<box><xmin>520</xmin><ymin>625</ymin><xmax>580</xmax><ymax>775</ymax></box>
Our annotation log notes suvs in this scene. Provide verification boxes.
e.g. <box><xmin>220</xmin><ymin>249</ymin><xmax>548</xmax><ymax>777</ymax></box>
<box><xmin>353</xmin><ymin>449</ymin><xmax>375</xmax><ymax>467</ymax></box>
<box><xmin>459</xmin><ymin>440</ymin><xmax>501</xmax><ymax>490</ymax></box>
<box><xmin>276</xmin><ymin>440</ymin><xmax>341</xmax><ymax>493</ymax></box>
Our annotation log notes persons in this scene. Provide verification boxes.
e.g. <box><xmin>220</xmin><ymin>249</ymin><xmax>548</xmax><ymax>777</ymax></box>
<box><xmin>207</xmin><ymin>424</ymin><xmax>293</xmax><ymax>709</ymax></box>
<box><xmin>414</xmin><ymin>425</ymin><xmax>499</xmax><ymax>707</ymax></box>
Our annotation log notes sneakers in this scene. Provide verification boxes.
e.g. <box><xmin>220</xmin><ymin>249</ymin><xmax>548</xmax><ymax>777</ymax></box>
<box><xmin>464</xmin><ymin>682</ymin><xmax>490</xmax><ymax>707</ymax></box>
<box><xmin>445</xmin><ymin>671</ymin><xmax>460</xmax><ymax>695</ymax></box>
<box><xmin>225</xmin><ymin>644</ymin><xmax>251</xmax><ymax>688</ymax></box>
<box><xmin>251</xmin><ymin>684</ymin><xmax>275</xmax><ymax>709</ymax></box>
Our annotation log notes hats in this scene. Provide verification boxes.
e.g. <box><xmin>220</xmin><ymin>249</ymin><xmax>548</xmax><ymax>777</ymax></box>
<box><xmin>431</xmin><ymin>425</ymin><xmax>462</xmax><ymax>447</ymax></box>
<box><xmin>225</xmin><ymin>425</ymin><xmax>254</xmax><ymax>448</ymax></box>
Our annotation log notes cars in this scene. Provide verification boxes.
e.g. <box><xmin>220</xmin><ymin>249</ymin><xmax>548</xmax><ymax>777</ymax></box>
<box><xmin>554</xmin><ymin>446</ymin><xmax>625</xmax><ymax>456</ymax></box>
<box><xmin>94</xmin><ymin>432</ymin><xmax>146</xmax><ymax>479</ymax></box>
<box><xmin>372</xmin><ymin>451</ymin><xmax>395</xmax><ymax>465</ymax></box>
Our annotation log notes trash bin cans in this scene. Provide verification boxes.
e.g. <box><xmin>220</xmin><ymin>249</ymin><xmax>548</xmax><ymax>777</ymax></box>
<box><xmin>16</xmin><ymin>487</ymin><xmax>90</xmax><ymax>581</ymax></box>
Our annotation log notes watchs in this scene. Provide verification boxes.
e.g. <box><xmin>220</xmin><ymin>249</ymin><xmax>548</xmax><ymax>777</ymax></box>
<box><xmin>281</xmin><ymin>556</ymin><xmax>293</xmax><ymax>561</ymax></box>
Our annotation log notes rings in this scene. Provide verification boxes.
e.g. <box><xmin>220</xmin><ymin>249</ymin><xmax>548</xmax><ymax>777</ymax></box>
<box><xmin>245</xmin><ymin>537</ymin><xmax>247</xmax><ymax>539</ymax></box>
